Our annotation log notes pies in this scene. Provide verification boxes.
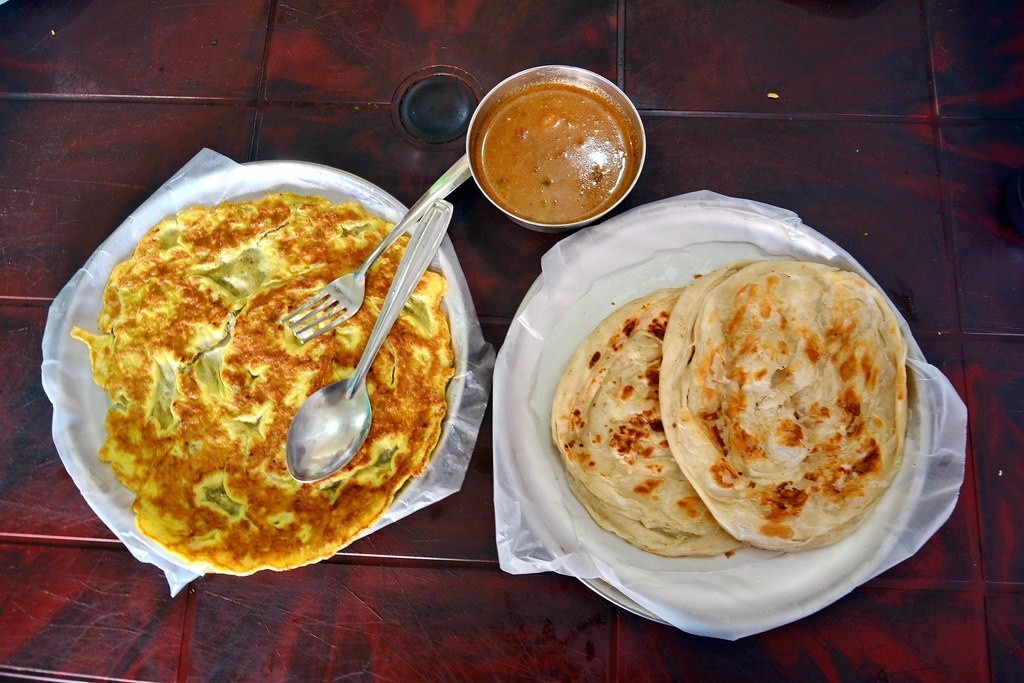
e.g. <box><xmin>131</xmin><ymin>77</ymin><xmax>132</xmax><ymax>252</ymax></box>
<box><xmin>550</xmin><ymin>286</ymin><xmax>754</xmax><ymax>558</ymax></box>
<box><xmin>70</xmin><ymin>197</ymin><xmax>458</xmax><ymax>576</ymax></box>
<box><xmin>656</xmin><ymin>257</ymin><xmax>907</xmax><ymax>551</ymax></box>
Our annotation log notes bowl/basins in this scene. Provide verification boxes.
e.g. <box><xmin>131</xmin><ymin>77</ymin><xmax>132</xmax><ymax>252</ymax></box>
<box><xmin>465</xmin><ymin>64</ymin><xmax>646</xmax><ymax>233</ymax></box>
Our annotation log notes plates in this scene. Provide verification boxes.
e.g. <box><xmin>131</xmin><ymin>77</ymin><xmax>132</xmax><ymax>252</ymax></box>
<box><xmin>503</xmin><ymin>205</ymin><xmax>931</xmax><ymax>632</ymax></box>
<box><xmin>56</xmin><ymin>161</ymin><xmax>469</xmax><ymax>578</ymax></box>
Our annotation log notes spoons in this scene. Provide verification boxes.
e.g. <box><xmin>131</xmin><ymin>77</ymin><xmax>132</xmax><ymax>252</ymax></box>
<box><xmin>285</xmin><ymin>199</ymin><xmax>455</xmax><ymax>485</ymax></box>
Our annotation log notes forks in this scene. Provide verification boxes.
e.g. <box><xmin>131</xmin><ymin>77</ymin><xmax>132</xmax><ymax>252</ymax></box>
<box><xmin>279</xmin><ymin>152</ymin><xmax>472</xmax><ymax>346</ymax></box>
